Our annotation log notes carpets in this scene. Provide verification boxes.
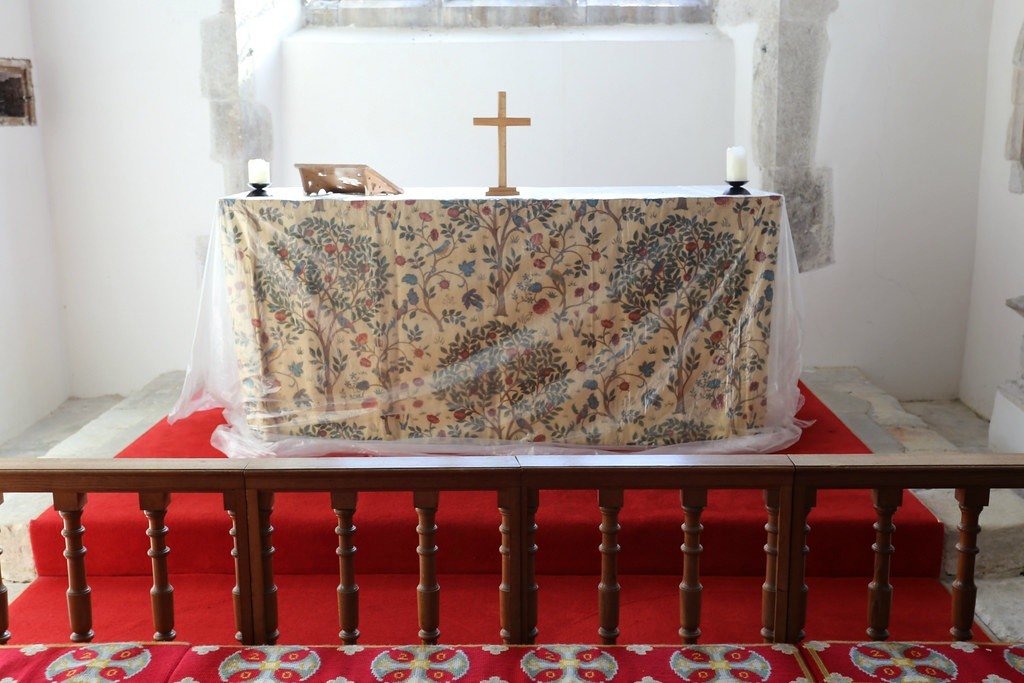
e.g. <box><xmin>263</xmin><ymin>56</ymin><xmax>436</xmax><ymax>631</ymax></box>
<box><xmin>1</xmin><ymin>381</ymin><xmax>1024</xmax><ymax>683</ymax></box>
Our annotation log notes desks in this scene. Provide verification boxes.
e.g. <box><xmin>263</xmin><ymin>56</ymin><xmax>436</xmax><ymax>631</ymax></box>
<box><xmin>219</xmin><ymin>187</ymin><xmax>784</xmax><ymax>447</ymax></box>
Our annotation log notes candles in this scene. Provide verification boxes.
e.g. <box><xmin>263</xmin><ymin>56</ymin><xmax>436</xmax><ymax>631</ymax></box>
<box><xmin>247</xmin><ymin>158</ymin><xmax>271</xmax><ymax>185</ymax></box>
<box><xmin>724</xmin><ymin>144</ymin><xmax>748</xmax><ymax>182</ymax></box>
